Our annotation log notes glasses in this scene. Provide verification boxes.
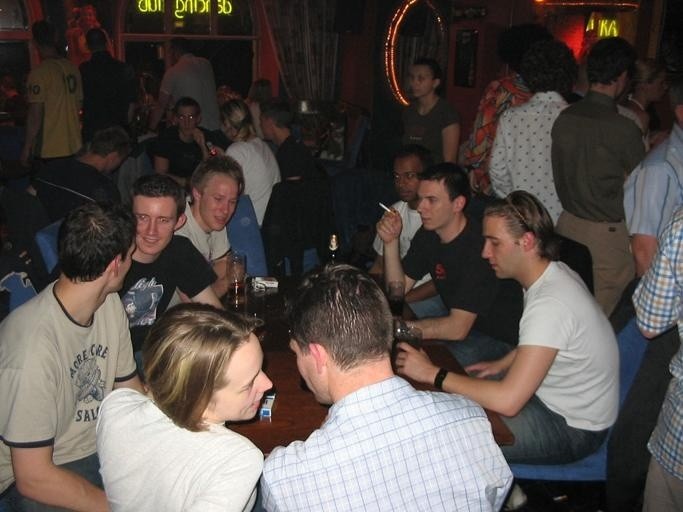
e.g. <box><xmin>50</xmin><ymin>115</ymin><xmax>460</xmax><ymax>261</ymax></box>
<box><xmin>178</xmin><ymin>115</ymin><xmax>194</xmax><ymax>121</ymax></box>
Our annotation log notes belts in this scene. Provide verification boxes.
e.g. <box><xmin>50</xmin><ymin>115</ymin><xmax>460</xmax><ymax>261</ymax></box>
<box><xmin>572</xmin><ymin>216</ymin><xmax>624</xmax><ymax>225</ymax></box>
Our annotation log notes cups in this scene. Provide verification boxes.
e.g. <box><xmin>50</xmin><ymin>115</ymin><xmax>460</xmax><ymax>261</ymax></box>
<box><xmin>394</xmin><ymin>326</ymin><xmax>424</xmax><ymax>352</ymax></box>
<box><xmin>388</xmin><ymin>280</ymin><xmax>404</xmax><ymax>317</ymax></box>
<box><xmin>227</xmin><ymin>284</ymin><xmax>245</xmax><ymax>314</ymax></box>
<box><xmin>226</xmin><ymin>251</ymin><xmax>246</xmax><ymax>283</ymax></box>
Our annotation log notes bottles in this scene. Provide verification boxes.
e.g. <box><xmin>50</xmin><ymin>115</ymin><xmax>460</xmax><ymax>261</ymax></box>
<box><xmin>327</xmin><ymin>234</ymin><xmax>342</xmax><ymax>265</ymax></box>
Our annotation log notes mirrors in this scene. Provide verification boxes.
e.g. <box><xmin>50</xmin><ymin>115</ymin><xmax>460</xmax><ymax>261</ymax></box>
<box><xmin>384</xmin><ymin>1</ymin><xmax>446</xmax><ymax>106</ymax></box>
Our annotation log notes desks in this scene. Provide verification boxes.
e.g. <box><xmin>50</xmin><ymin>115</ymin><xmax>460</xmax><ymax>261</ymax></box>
<box><xmin>227</xmin><ymin>292</ymin><xmax>517</xmax><ymax>457</ymax></box>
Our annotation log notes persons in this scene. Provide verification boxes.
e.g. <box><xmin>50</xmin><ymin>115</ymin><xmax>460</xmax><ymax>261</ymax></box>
<box><xmin>370</xmin><ymin>23</ymin><xmax>683</xmax><ymax>511</ymax></box>
<box><xmin>96</xmin><ymin>302</ymin><xmax>274</xmax><ymax>512</ymax></box>
<box><xmin>259</xmin><ymin>263</ymin><xmax>514</xmax><ymax>512</ymax></box>
<box><xmin>1</xmin><ymin>21</ymin><xmax>312</xmax><ymax>384</ymax></box>
<box><xmin>0</xmin><ymin>200</ymin><xmax>145</xmax><ymax>512</ymax></box>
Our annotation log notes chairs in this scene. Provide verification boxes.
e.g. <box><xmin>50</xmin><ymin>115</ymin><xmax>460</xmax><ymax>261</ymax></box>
<box><xmin>33</xmin><ymin>216</ymin><xmax>70</xmax><ymax>276</ymax></box>
<box><xmin>507</xmin><ymin>276</ymin><xmax>680</xmax><ymax>512</ymax></box>
<box><xmin>226</xmin><ymin>193</ymin><xmax>270</xmax><ymax>279</ymax></box>
<box><xmin>0</xmin><ymin>271</ymin><xmax>37</xmax><ymax>310</ymax></box>
<box><xmin>553</xmin><ymin>233</ymin><xmax>594</xmax><ymax>291</ymax></box>
